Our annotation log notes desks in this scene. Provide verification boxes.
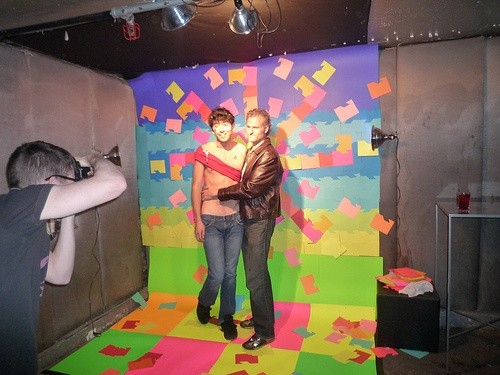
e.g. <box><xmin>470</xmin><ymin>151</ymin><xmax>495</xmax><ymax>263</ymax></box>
<box><xmin>434</xmin><ymin>199</ymin><xmax>500</xmax><ymax>357</ymax></box>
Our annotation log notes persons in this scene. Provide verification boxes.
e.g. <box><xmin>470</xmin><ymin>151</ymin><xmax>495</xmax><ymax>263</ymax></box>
<box><xmin>190</xmin><ymin>107</ymin><xmax>249</xmax><ymax>341</ymax></box>
<box><xmin>0</xmin><ymin>139</ymin><xmax>127</xmax><ymax>374</ymax></box>
<box><xmin>200</xmin><ymin>109</ymin><xmax>284</xmax><ymax>349</ymax></box>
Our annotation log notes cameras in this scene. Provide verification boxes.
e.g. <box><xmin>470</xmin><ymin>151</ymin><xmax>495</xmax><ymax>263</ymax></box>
<box><xmin>74</xmin><ymin>161</ymin><xmax>91</xmax><ymax>182</ymax></box>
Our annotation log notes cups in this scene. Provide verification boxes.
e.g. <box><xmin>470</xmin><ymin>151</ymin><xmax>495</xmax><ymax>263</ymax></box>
<box><xmin>456</xmin><ymin>191</ymin><xmax>471</xmax><ymax>211</ymax></box>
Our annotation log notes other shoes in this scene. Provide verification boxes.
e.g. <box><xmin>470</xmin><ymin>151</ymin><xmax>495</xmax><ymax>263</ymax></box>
<box><xmin>240</xmin><ymin>318</ymin><xmax>254</xmax><ymax>329</ymax></box>
<box><xmin>219</xmin><ymin>316</ymin><xmax>238</xmax><ymax>340</ymax></box>
<box><xmin>197</xmin><ymin>303</ymin><xmax>212</xmax><ymax>324</ymax></box>
<box><xmin>242</xmin><ymin>333</ymin><xmax>275</xmax><ymax>350</ymax></box>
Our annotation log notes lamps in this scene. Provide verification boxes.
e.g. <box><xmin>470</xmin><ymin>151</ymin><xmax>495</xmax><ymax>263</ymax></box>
<box><xmin>91</xmin><ymin>142</ymin><xmax>123</xmax><ymax>171</ymax></box>
<box><xmin>226</xmin><ymin>0</ymin><xmax>258</xmax><ymax>37</ymax></box>
<box><xmin>156</xmin><ymin>4</ymin><xmax>198</xmax><ymax>33</ymax></box>
<box><xmin>371</xmin><ymin>126</ymin><xmax>400</xmax><ymax>152</ymax></box>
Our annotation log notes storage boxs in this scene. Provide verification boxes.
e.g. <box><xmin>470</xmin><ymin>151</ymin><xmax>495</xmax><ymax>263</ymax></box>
<box><xmin>375</xmin><ymin>273</ymin><xmax>441</xmax><ymax>353</ymax></box>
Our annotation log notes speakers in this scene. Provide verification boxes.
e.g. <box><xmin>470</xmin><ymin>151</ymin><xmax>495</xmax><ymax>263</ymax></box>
<box><xmin>375</xmin><ymin>279</ymin><xmax>440</xmax><ymax>353</ymax></box>
